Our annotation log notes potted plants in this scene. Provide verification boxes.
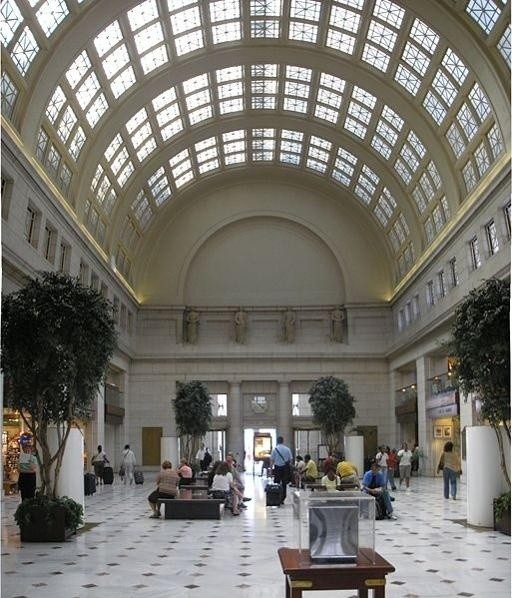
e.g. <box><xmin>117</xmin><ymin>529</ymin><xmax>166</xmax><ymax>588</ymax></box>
<box><xmin>2</xmin><ymin>270</ymin><xmax>117</xmax><ymax>543</ymax></box>
<box><xmin>434</xmin><ymin>274</ymin><xmax>510</xmax><ymax>534</ymax></box>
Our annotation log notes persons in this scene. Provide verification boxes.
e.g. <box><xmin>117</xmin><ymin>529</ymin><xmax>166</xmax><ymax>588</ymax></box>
<box><xmin>91</xmin><ymin>444</ymin><xmax>111</xmax><ymax>485</ymax></box>
<box><xmin>281</xmin><ymin>306</ymin><xmax>297</xmax><ymax>343</ymax></box>
<box><xmin>120</xmin><ymin>444</ymin><xmax>136</xmax><ymax>485</ymax></box>
<box><xmin>436</xmin><ymin>441</ymin><xmax>461</xmax><ymax>501</ymax></box>
<box><xmin>233</xmin><ymin>306</ymin><xmax>249</xmax><ymax>345</ymax></box>
<box><xmin>147</xmin><ymin>439</ymin><xmax>419</xmax><ymax>524</ymax></box>
<box><xmin>18</xmin><ymin>445</ymin><xmax>37</xmax><ymax>501</ymax></box>
<box><xmin>185</xmin><ymin>306</ymin><xmax>200</xmax><ymax>343</ymax></box>
<box><xmin>330</xmin><ymin>303</ymin><xmax>345</xmax><ymax>343</ymax></box>
<box><xmin>268</xmin><ymin>436</ymin><xmax>292</xmax><ymax>504</ymax></box>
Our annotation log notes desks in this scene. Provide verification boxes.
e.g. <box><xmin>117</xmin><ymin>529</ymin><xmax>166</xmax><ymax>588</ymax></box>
<box><xmin>277</xmin><ymin>546</ymin><xmax>395</xmax><ymax>598</ymax></box>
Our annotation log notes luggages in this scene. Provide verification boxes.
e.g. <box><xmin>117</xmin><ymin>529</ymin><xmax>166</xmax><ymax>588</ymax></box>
<box><xmin>265</xmin><ymin>467</ymin><xmax>281</xmax><ymax>507</ymax></box>
<box><xmin>84</xmin><ymin>473</ymin><xmax>96</xmax><ymax>496</ymax></box>
<box><xmin>134</xmin><ymin>467</ymin><xmax>144</xmax><ymax>485</ymax></box>
<box><xmin>103</xmin><ymin>467</ymin><xmax>113</xmax><ymax>484</ymax></box>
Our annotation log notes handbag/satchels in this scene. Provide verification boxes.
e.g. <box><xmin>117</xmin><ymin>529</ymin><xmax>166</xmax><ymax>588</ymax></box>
<box><xmin>290</xmin><ymin>471</ymin><xmax>295</xmax><ymax>484</ymax></box>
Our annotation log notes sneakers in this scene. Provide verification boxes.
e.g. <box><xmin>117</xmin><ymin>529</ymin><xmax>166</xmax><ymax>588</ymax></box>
<box><xmin>233</xmin><ymin>498</ymin><xmax>251</xmax><ymax>515</ymax></box>
<box><xmin>391</xmin><ymin>484</ymin><xmax>413</xmax><ymax>492</ymax></box>
<box><xmin>149</xmin><ymin>512</ymin><xmax>161</xmax><ymax>518</ymax></box>
<box><xmin>388</xmin><ymin>514</ymin><xmax>397</xmax><ymax>520</ymax></box>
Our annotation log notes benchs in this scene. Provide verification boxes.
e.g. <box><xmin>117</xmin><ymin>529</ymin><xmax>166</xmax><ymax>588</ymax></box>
<box><xmin>150</xmin><ymin>468</ymin><xmax>395</xmax><ymax>519</ymax></box>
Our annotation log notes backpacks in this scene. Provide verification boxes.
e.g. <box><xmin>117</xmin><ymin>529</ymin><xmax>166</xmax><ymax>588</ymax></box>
<box><xmin>375</xmin><ymin>497</ymin><xmax>385</xmax><ymax>519</ymax></box>
